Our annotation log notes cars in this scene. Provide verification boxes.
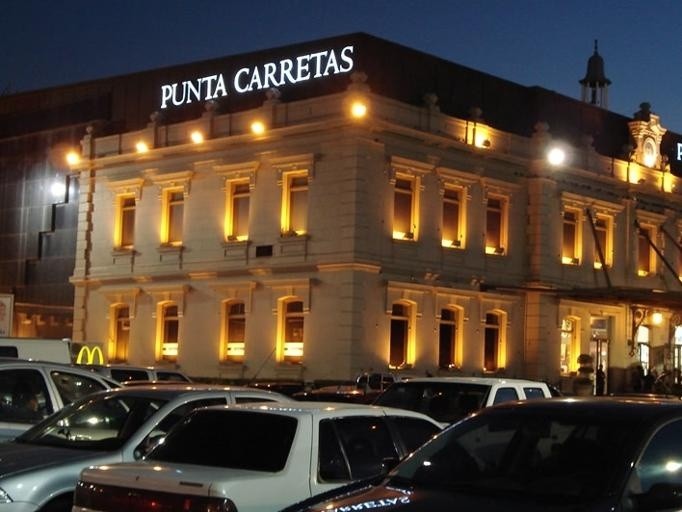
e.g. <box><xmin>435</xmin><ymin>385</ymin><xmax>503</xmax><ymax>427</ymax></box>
<box><xmin>72</xmin><ymin>401</ymin><xmax>445</xmax><ymax>512</ymax></box>
<box><xmin>0</xmin><ymin>382</ymin><xmax>295</xmax><ymax>512</ymax></box>
<box><xmin>277</xmin><ymin>394</ymin><xmax>682</xmax><ymax>512</ymax></box>
<box><xmin>75</xmin><ymin>364</ymin><xmax>193</xmax><ymax>384</ymax></box>
<box><xmin>369</xmin><ymin>376</ymin><xmax>552</xmax><ymax>425</ymax></box>
<box><xmin>0</xmin><ymin>359</ymin><xmax>161</xmax><ymax>449</ymax></box>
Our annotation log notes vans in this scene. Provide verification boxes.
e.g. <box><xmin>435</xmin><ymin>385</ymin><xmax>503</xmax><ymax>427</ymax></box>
<box><xmin>0</xmin><ymin>336</ymin><xmax>74</xmax><ymax>366</ymax></box>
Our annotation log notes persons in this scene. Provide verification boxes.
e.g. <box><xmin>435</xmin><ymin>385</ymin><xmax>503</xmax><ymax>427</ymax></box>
<box><xmin>12</xmin><ymin>382</ymin><xmax>42</xmax><ymax>412</ymax></box>
<box><xmin>357</xmin><ymin>367</ymin><xmax>375</xmax><ymax>395</ymax></box>
<box><xmin>631</xmin><ymin>365</ymin><xmax>682</xmax><ymax>397</ymax></box>
<box><xmin>596</xmin><ymin>364</ymin><xmax>605</xmax><ymax>396</ymax></box>
